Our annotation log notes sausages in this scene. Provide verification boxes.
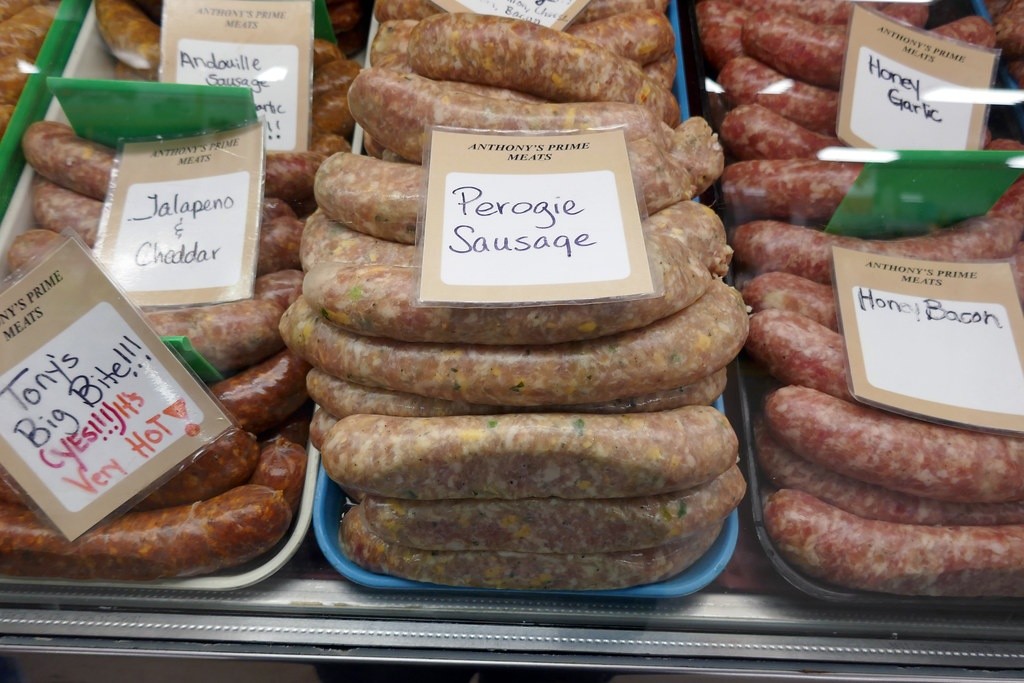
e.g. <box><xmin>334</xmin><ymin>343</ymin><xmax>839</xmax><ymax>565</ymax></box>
<box><xmin>0</xmin><ymin>0</ymin><xmax>1024</xmax><ymax>598</ymax></box>
